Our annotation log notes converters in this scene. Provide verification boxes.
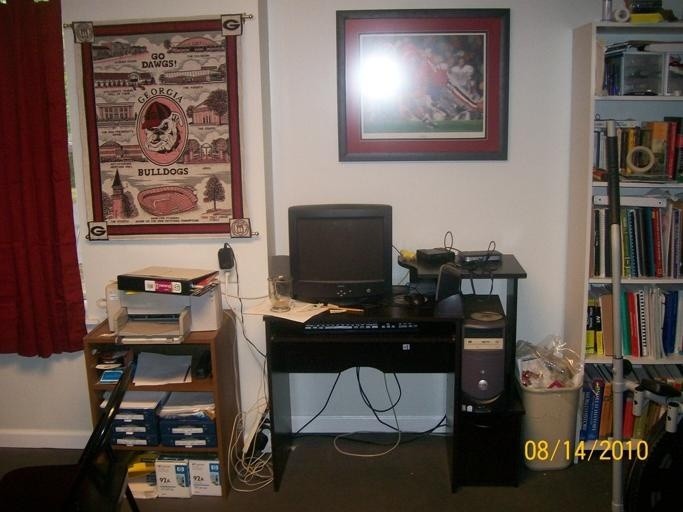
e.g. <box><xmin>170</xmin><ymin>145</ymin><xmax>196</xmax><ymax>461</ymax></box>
<box><xmin>218</xmin><ymin>249</ymin><xmax>234</xmax><ymax>270</ymax></box>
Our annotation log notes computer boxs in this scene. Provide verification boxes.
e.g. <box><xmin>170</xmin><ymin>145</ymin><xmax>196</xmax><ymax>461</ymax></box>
<box><xmin>462</xmin><ymin>293</ymin><xmax>506</xmax><ymax>404</ymax></box>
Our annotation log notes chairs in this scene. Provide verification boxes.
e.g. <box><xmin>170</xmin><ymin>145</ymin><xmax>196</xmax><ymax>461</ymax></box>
<box><xmin>0</xmin><ymin>360</ymin><xmax>137</xmax><ymax>511</ymax></box>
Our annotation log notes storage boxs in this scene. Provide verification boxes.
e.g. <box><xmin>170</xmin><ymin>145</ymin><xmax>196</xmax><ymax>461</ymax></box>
<box><xmin>122</xmin><ymin>453</ymin><xmax>224</xmax><ymax>499</ymax></box>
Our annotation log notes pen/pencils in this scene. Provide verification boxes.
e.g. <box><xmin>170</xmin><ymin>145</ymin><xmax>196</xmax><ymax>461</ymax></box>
<box><xmin>324</xmin><ymin>306</ymin><xmax>365</xmax><ymax>312</ymax></box>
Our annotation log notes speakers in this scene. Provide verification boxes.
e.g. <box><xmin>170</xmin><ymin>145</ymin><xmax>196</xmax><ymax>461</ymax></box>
<box><xmin>270</xmin><ymin>256</ymin><xmax>291</xmax><ymax>297</ymax></box>
<box><xmin>434</xmin><ymin>262</ymin><xmax>464</xmax><ymax>321</ymax></box>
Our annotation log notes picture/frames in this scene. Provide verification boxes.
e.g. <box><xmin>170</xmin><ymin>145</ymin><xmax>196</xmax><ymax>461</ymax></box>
<box><xmin>62</xmin><ymin>11</ymin><xmax>256</xmax><ymax>242</ymax></box>
<box><xmin>334</xmin><ymin>6</ymin><xmax>510</xmax><ymax>162</ymax></box>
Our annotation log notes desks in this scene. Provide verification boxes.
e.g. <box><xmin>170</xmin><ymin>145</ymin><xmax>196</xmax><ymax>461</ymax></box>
<box><xmin>261</xmin><ymin>289</ymin><xmax>524</xmax><ymax>493</ymax></box>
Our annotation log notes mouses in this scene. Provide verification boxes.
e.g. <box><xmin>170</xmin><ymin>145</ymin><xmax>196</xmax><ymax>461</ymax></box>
<box><xmin>408</xmin><ymin>292</ymin><xmax>425</xmax><ymax>308</ymax></box>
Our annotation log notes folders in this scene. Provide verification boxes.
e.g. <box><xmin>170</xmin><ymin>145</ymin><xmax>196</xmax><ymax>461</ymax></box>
<box><xmin>117</xmin><ymin>266</ymin><xmax>219</xmax><ymax>294</ymax></box>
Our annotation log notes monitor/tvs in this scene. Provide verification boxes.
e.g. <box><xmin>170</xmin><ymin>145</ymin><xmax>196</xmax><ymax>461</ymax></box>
<box><xmin>287</xmin><ymin>203</ymin><xmax>392</xmax><ymax>305</ymax></box>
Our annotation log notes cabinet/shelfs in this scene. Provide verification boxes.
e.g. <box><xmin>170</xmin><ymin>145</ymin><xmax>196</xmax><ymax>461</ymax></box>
<box><xmin>562</xmin><ymin>20</ymin><xmax>683</xmax><ymax>462</ymax></box>
<box><xmin>83</xmin><ymin>310</ymin><xmax>246</xmax><ymax>503</ymax></box>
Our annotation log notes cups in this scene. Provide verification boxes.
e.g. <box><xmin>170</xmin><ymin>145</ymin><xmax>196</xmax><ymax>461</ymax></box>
<box><xmin>266</xmin><ymin>276</ymin><xmax>292</xmax><ymax>314</ymax></box>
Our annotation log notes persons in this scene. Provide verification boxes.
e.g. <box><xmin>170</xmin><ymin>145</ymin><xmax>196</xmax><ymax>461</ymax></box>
<box><xmin>360</xmin><ymin>35</ymin><xmax>484</xmax><ymax>131</ymax></box>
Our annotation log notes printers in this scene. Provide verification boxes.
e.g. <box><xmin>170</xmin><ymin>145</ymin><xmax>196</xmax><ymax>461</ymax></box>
<box><xmin>104</xmin><ymin>279</ymin><xmax>223</xmax><ymax>346</ymax></box>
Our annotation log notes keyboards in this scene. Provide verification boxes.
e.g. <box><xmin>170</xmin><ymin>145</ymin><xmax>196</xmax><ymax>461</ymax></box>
<box><xmin>305</xmin><ymin>320</ymin><xmax>418</xmax><ymax>337</ymax></box>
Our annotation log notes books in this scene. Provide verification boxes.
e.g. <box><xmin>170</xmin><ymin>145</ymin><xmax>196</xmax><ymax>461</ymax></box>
<box><xmin>580</xmin><ymin>116</ymin><xmax>682</xmax><ymax>442</ymax></box>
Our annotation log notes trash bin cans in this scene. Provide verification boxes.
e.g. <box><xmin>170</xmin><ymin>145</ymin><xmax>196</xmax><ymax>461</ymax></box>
<box><xmin>518</xmin><ymin>349</ymin><xmax>581</xmax><ymax>472</ymax></box>
